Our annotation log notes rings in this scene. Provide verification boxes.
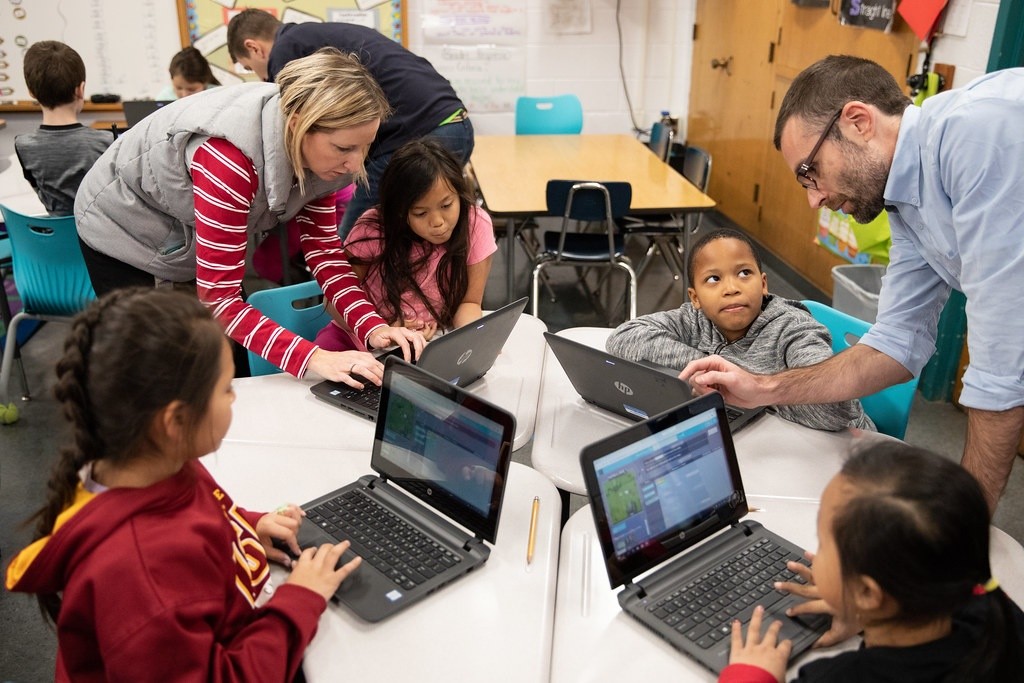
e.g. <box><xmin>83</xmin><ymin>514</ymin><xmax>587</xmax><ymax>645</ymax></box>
<box><xmin>350</xmin><ymin>364</ymin><xmax>356</xmax><ymax>372</ymax></box>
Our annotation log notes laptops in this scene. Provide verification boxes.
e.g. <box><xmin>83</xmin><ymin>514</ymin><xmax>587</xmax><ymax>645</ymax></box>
<box><xmin>122</xmin><ymin>100</ymin><xmax>176</xmax><ymax>128</ymax></box>
<box><xmin>310</xmin><ymin>296</ymin><xmax>529</xmax><ymax>423</ymax></box>
<box><xmin>543</xmin><ymin>332</ymin><xmax>767</xmax><ymax>436</ymax></box>
<box><xmin>579</xmin><ymin>390</ymin><xmax>832</xmax><ymax>677</ymax></box>
<box><xmin>272</xmin><ymin>355</ymin><xmax>516</xmax><ymax>622</ymax></box>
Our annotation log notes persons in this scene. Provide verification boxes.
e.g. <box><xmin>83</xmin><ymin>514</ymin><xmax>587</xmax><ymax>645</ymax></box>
<box><xmin>606</xmin><ymin>229</ymin><xmax>877</xmax><ymax>433</ymax></box>
<box><xmin>679</xmin><ymin>56</ymin><xmax>1024</xmax><ymax>522</ymax></box>
<box><xmin>715</xmin><ymin>440</ymin><xmax>1024</xmax><ymax>683</ymax></box>
<box><xmin>254</xmin><ymin>182</ymin><xmax>357</xmax><ymax>299</ymax></box>
<box><xmin>74</xmin><ymin>46</ymin><xmax>427</xmax><ymax>389</ymax></box>
<box><xmin>6</xmin><ymin>285</ymin><xmax>363</xmax><ymax>682</ymax></box>
<box><xmin>169</xmin><ymin>47</ymin><xmax>221</xmax><ymax>98</ymax></box>
<box><xmin>229</xmin><ymin>9</ymin><xmax>474</xmax><ymax>244</ymax></box>
<box><xmin>312</xmin><ymin>138</ymin><xmax>499</xmax><ymax>351</ymax></box>
<box><xmin>15</xmin><ymin>40</ymin><xmax>154</xmax><ymax>296</ymax></box>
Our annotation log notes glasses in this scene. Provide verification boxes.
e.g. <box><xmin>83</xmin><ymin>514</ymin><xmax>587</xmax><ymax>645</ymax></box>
<box><xmin>796</xmin><ymin>105</ymin><xmax>845</xmax><ymax>191</ymax></box>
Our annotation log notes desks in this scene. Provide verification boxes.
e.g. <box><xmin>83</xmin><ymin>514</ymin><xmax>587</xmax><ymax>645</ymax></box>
<box><xmin>92</xmin><ymin>119</ymin><xmax>128</xmax><ymax>142</ymax></box>
<box><xmin>201</xmin><ymin>308</ymin><xmax>1024</xmax><ymax>683</ymax></box>
<box><xmin>468</xmin><ymin>132</ymin><xmax>716</xmax><ymax>307</ymax></box>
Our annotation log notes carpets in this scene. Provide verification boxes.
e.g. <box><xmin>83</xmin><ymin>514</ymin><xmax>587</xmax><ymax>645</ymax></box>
<box><xmin>0</xmin><ymin>271</ymin><xmax>43</xmax><ymax>355</ymax></box>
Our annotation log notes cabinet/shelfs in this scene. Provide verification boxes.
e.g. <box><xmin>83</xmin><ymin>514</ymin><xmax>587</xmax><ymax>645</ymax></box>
<box><xmin>687</xmin><ymin>0</ymin><xmax>919</xmax><ymax>297</ymax></box>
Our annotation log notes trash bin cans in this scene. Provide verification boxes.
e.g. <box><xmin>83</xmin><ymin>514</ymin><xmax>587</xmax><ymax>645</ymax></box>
<box><xmin>831</xmin><ymin>264</ymin><xmax>886</xmax><ymax>345</ymax></box>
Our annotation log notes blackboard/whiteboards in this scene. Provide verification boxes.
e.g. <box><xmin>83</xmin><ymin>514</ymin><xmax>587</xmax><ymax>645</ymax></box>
<box><xmin>0</xmin><ymin>0</ymin><xmax>194</xmax><ymax>112</ymax></box>
<box><xmin>840</xmin><ymin>0</ymin><xmax>898</xmax><ymax>30</ymax></box>
<box><xmin>176</xmin><ymin>0</ymin><xmax>407</xmax><ymax>91</ymax></box>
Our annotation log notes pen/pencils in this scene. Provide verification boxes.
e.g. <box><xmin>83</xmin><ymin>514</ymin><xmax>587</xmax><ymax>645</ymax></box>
<box><xmin>527</xmin><ymin>496</ymin><xmax>539</xmax><ymax>565</ymax></box>
<box><xmin>748</xmin><ymin>508</ymin><xmax>760</xmax><ymax>512</ymax></box>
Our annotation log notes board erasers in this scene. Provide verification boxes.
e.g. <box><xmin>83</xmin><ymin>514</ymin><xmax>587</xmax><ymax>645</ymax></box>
<box><xmin>90</xmin><ymin>95</ymin><xmax>120</xmax><ymax>103</ymax></box>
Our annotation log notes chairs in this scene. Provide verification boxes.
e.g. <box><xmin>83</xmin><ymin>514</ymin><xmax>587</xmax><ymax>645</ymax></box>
<box><xmin>0</xmin><ymin>201</ymin><xmax>99</xmax><ymax>426</ymax></box>
<box><xmin>465</xmin><ymin>93</ymin><xmax>712</xmax><ymax>327</ymax></box>
<box><xmin>796</xmin><ymin>300</ymin><xmax>922</xmax><ymax>439</ymax></box>
<box><xmin>247</xmin><ymin>276</ymin><xmax>335</xmax><ymax>374</ymax></box>
